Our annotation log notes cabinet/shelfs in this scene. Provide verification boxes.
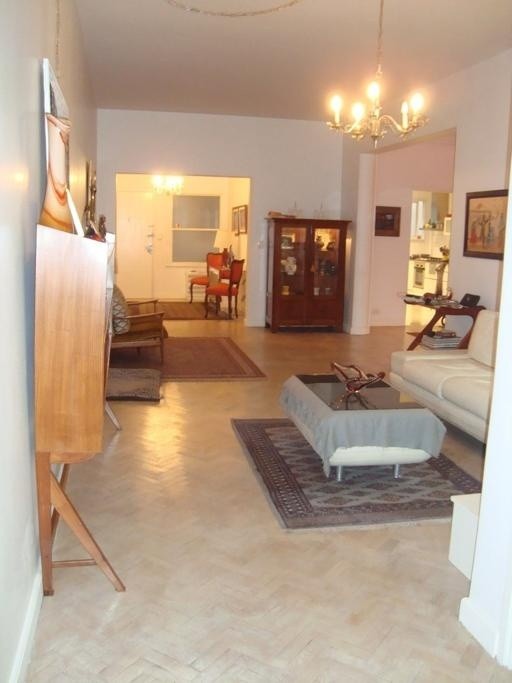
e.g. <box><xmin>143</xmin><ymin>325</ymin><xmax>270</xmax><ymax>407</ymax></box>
<box><xmin>402</xmin><ymin>295</ymin><xmax>487</xmax><ymax>352</ymax></box>
<box><xmin>264</xmin><ymin>217</ymin><xmax>352</xmax><ymax>334</ymax></box>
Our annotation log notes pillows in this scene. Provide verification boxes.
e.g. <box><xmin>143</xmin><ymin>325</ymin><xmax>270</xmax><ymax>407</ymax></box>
<box><xmin>106</xmin><ymin>367</ymin><xmax>161</xmax><ymax>401</ymax></box>
<box><xmin>113</xmin><ymin>283</ymin><xmax>132</xmax><ymax>334</ymax></box>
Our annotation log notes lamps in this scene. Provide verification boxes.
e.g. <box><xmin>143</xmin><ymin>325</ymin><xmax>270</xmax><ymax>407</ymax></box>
<box><xmin>324</xmin><ymin>0</ymin><xmax>431</xmax><ymax>150</ymax></box>
<box><xmin>213</xmin><ymin>230</ymin><xmax>235</xmax><ymax>268</ymax></box>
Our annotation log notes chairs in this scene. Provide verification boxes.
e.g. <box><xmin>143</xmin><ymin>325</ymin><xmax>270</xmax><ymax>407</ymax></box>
<box><xmin>111</xmin><ymin>299</ymin><xmax>168</xmax><ymax>366</ymax></box>
<box><xmin>204</xmin><ymin>259</ymin><xmax>244</xmax><ymax>319</ymax></box>
<box><xmin>189</xmin><ymin>252</ymin><xmax>224</xmax><ymax>304</ymax></box>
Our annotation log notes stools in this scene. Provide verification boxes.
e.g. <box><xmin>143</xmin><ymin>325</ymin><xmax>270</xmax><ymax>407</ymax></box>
<box><xmin>448</xmin><ymin>493</ymin><xmax>481</xmax><ymax>581</ymax></box>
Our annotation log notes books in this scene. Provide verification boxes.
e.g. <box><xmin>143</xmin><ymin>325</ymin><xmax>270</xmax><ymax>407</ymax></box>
<box><xmin>420</xmin><ymin>332</ymin><xmax>461</xmax><ymax>349</ymax></box>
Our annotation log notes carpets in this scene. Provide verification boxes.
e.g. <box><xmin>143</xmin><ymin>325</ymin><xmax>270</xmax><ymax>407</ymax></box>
<box><xmin>126</xmin><ymin>302</ymin><xmax>233</xmax><ymax>320</ymax></box>
<box><xmin>109</xmin><ymin>337</ymin><xmax>268</xmax><ymax>381</ymax></box>
<box><xmin>231</xmin><ymin>418</ymin><xmax>482</xmax><ymax>530</ymax></box>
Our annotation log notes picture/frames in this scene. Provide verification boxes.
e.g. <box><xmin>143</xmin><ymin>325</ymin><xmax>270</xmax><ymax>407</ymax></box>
<box><xmin>463</xmin><ymin>189</ymin><xmax>509</xmax><ymax>261</ymax></box>
<box><xmin>375</xmin><ymin>205</ymin><xmax>401</xmax><ymax>237</ymax></box>
<box><xmin>239</xmin><ymin>204</ymin><xmax>247</xmax><ymax>233</ymax></box>
<box><xmin>232</xmin><ymin>206</ymin><xmax>239</xmax><ymax>236</ymax></box>
<box><xmin>43</xmin><ymin>57</ymin><xmax>70</xmax><ymax>175</ymax></box>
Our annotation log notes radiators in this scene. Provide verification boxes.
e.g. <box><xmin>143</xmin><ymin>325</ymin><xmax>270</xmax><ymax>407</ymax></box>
<box><xmin>185</xmin><ymin>268</ymin><xmax>218</xmax><ymax>303</ymax></box>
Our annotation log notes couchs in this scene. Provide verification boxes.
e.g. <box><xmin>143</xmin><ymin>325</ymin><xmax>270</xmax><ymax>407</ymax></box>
<box><xmin>389</xmin><ymin>310</ymin><xmax>499</xmax><ymax>445</ymax></box>
